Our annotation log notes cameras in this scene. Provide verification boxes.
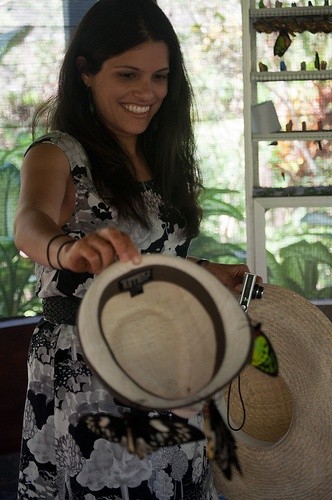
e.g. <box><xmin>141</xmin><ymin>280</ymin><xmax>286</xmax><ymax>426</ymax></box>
<box><xmin>239</xmin><ymin>272</ymin><xmax>265</xmax><ymax>313</ymax></box>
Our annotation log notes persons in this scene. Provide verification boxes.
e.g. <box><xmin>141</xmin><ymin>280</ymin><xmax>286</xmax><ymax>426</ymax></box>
<box><xmin>13</xmin><ymin>0</ymin><xmax>262</xmax><ymax>500</ymax></box>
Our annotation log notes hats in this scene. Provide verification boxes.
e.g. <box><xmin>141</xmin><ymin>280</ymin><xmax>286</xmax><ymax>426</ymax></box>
<box><xmin>77</xmin><ymin>253</ymin><xmax>255</xmax><ymax>409</ymax></box>
<box><xmin>202</xmin><ymin>282</ymin><xmax>332</xmax><ymax>500</ymax></box>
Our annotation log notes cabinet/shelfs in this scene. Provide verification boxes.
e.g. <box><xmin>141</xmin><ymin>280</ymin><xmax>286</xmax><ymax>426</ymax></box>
<box><xmin>241</xmin><ymin>0</ymin><xmax>331</xmax><ymax>305</ymax></box>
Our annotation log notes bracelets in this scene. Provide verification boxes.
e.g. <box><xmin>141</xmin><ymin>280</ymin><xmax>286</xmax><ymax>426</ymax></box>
<box><xmin>46</xmin><ymin>231</ymin><xmax>76</xmax><ymax>271</ymax></box>
<box><xmin>196</xmin><ymin>257</ymin><xmax>209</xmax><ymax>266</ymax></box>
